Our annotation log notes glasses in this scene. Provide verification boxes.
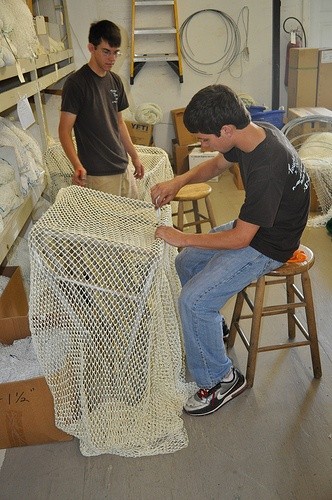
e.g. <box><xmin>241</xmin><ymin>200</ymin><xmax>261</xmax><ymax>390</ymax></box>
<box><xmin>95</xmin><ymin>46</ymin><xmax>121</xmax><ymax>57</ymax></box>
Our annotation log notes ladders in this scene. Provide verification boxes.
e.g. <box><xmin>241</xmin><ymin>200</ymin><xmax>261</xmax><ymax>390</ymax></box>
<box><xmin>129</xmin><ymin>0</ymin><xmax>184</xmax><ymax>85</ymax></box>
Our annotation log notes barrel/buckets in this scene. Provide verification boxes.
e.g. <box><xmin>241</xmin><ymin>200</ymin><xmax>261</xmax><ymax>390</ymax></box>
<box><xmin>244</xmin><ymin>106</ymin><xmax>286</xmax><ymax>132</ymax></box>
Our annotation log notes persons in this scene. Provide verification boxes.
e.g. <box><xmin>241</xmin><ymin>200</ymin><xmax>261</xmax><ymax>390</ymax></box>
<box><xmin>150</xmin><ymin>84</ymin><xmax>311</xmax><ymax>416</ymax></box>
<box><xmin>58</xmin><ymin>20</ymin><xmax>144</xmax><ymax>198</ymax></box>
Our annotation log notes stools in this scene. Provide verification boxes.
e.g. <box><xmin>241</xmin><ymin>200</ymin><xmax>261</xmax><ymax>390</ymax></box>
<box><xmin>168</xmin><ymin>183</ymin><xmax>215</xmax><ymax>253</ymax></box>
<box><xmin>228</xmin><ymin>244</ymin><xmax>322</xmax><ymax>388</ymax></box>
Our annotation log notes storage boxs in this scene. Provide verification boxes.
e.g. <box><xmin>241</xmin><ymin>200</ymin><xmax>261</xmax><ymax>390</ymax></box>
<box><xmin>287</xmin><ymin>48</ymin><xmax>318</xmax><ymax>108</ymax></box>
<box><xmin>317</xmin><ymin>48</ymin><xmax>332</xmax><ymax>108</ymax></box>
<box><xmin>172</xmin><ymin>108</ymin><xmax>221</xmax><ymax>185</ymax></box>
<box><xmin>288</xmin><ymin>106</ymin><xmax>332</xmax><ymax>145</ymax></box>
<box><xmin>0</xmin><ymin>265</ymin><xmax>30</xmax><ymax>346</ymax></box>
<box><xmin>124</xmin><ymin>118</ymin><xmax>154</xmax><ymax>145</ymax></box>
<box><xmin>0</xmin><ymin>318</ymin><xmax>77</xmax><ymax>449</ymax></box>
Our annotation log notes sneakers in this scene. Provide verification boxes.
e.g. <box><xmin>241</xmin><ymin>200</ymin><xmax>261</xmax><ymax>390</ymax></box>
<box><xmin>222</xmin><ymin>318</ymin><xmax>230</xmax><ymax>339</ymax></box>
<box><xmin>183</xmin><ymin>368</ymin><xmax>247</xmax><ymax>415</ymax></box>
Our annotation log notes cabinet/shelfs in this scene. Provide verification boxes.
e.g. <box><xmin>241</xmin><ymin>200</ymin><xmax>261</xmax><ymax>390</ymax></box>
<box><xmin>0</xmin><ymin>0</ymin><xmax>76</xmax><ymax>265</ymax></box>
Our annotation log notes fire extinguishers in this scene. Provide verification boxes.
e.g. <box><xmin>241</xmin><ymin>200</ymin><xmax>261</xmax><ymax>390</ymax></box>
<box><xmin>283</xmin><ymin>27</ymin><xmax>302</xmax><ymax>87</ymax></box>
